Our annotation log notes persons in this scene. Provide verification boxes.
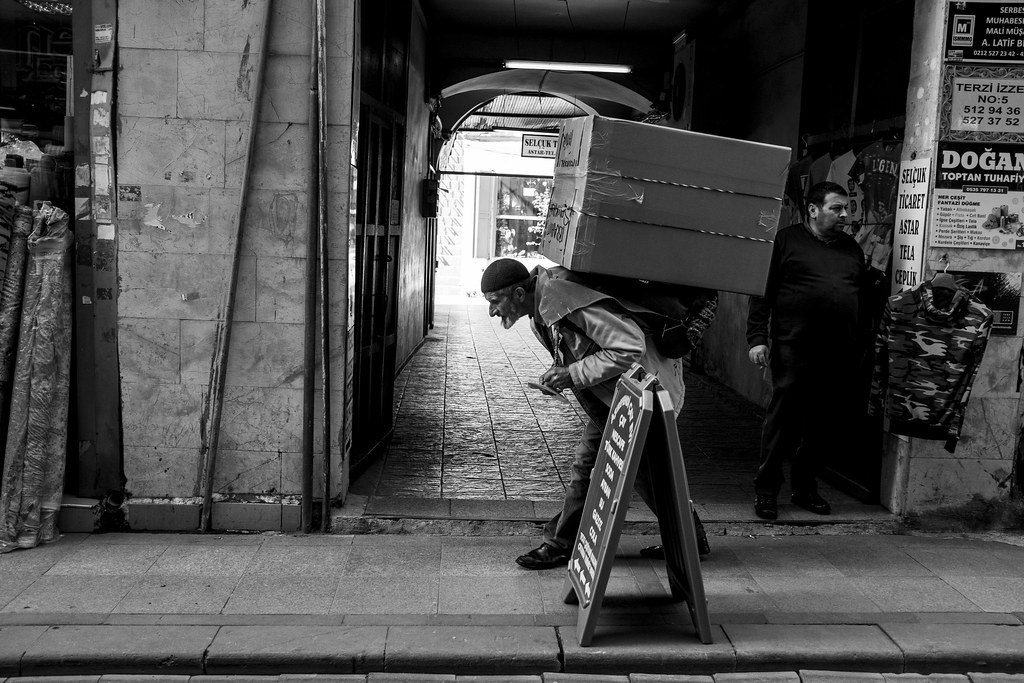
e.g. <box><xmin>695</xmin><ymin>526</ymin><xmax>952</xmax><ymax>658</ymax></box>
<box><xmin>747</xmin><ymin>181</ymin><xmax>872</xmax><ymax>519</ymax></box>
<box><xmin>481</xmin><ymin>258</ymin><xmax>711</xmax><ymax>569</ymax></box>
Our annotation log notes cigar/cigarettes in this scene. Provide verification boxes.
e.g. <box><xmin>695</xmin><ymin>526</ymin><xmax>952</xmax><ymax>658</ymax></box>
<box><xmin>759</xmin><ymin>365</ymin><xmax>764</xmax><ymax>370</ymax></box>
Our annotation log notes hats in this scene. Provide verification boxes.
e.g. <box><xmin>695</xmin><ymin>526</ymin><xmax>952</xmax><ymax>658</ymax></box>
<box><xmin>480</xmin><ymin>258</ymin><xmax>530</xmax><ymax>293</ymax></box>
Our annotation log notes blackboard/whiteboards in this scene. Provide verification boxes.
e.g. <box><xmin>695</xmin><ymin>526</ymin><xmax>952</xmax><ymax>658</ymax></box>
<box><xmin>561</xmin><ymin>362</ymin><xmax>714</xmax><ymax>611</ymax></box>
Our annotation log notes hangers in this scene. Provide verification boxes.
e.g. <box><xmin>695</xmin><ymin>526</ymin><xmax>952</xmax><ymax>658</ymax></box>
<box><xmin>931</xmin><ymin>257</ymin><xmax>960</xmax><ymax>292</ymax></box>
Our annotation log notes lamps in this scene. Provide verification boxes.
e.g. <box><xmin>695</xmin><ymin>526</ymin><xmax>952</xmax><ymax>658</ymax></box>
<box><xmin>501</xmin><ymin>59</ymin><xmax>633</xmax><ymax>75</ymax></box>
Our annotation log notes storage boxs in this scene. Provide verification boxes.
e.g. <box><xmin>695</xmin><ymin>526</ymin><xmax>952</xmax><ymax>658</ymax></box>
<box><xmin>537</xmin><ymin>112</ymin><xmax>794</xmax><ymax>298</ymax></box>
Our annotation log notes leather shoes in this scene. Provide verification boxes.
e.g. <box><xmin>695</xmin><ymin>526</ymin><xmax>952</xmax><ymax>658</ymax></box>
<box><xmin>791</xmin><ymin>489</ymin><xmax>831</xmax><ymax>514</ymax></box>
<box><xmin>754</xmin><ymin>491</ymin><xmax>777</xmax><ymax>520</ymax></box>
<box><xmin>639</xmin><ymin>535</ymin><xmax>711</xmax><ymax>560</ymax></box>
<box><xmin>516</xmin><ymin>540</ymin><xmax>572</xmax><ymax>570</ymax></box>
